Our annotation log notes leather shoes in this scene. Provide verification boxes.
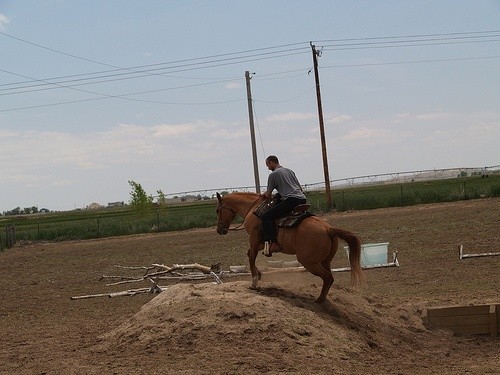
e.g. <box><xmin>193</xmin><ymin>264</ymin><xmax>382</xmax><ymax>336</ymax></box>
<box><xmin>262</xmin><ymin>242</ymin><xmax>282</xmax><ymax>254</ymax></box>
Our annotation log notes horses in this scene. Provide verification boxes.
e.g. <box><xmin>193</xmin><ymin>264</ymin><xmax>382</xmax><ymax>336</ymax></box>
<box><xmin>215</xmin><ymin>192</ymin><xmax>363</xmax><ymax>304</ymax></box>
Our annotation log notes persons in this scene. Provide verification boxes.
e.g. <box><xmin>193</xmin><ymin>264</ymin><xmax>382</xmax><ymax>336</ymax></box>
<box><xmin>261</xmin><ymin>155</ymin><xmax>307</xmax><ymax>255</ymax></box>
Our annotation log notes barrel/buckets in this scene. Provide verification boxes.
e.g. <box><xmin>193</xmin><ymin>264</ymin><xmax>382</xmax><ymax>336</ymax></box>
<box><xmin>343</xmin><ymin>242</ymin><xmax>389</xmax><ymax>266</ymax></box>
